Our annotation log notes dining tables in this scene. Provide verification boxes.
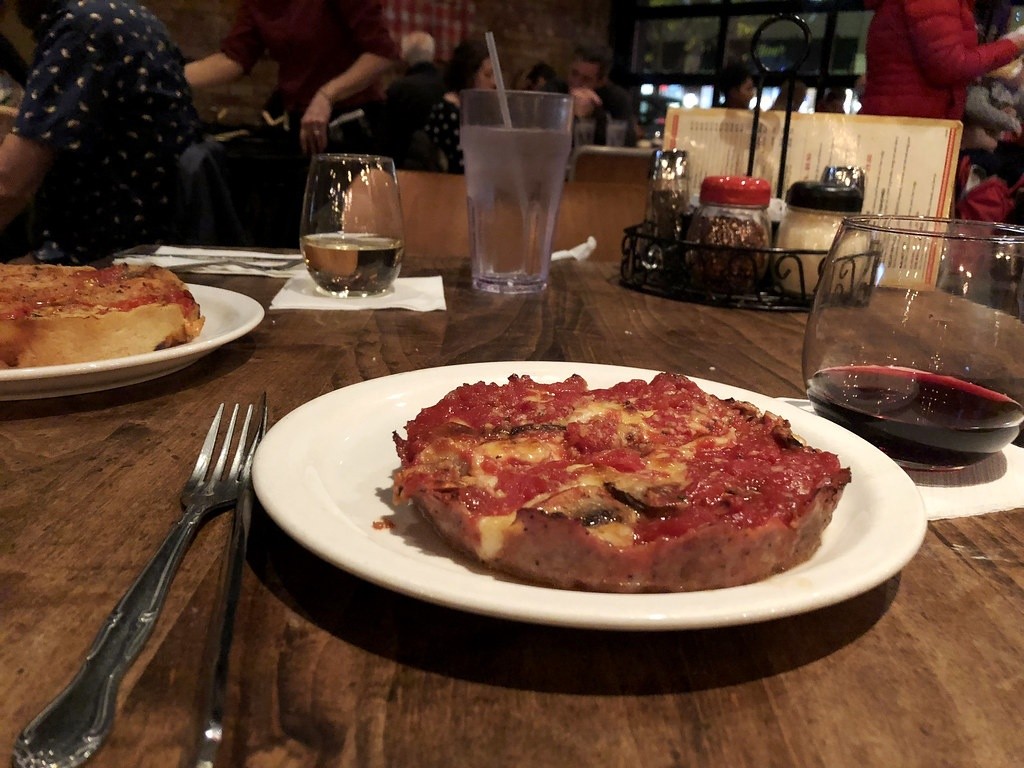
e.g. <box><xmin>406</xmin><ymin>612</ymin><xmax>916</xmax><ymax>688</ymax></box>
<box><xmin>0</xmin><ymin>244</ymin><xmax>1024</xmax><ymax>768</ymax></box>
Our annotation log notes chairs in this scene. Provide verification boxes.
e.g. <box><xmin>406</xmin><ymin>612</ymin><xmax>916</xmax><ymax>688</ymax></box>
<box><xmin>343</xmin><ymin>167</ymin><xmax>648</xmax><ymax>260</ymax></box>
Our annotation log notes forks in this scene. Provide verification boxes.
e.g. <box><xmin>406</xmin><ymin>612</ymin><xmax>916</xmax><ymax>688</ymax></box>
<box><xmin>9</xmin><ymin>400</ymin><xmax>268</xmax><ymax>768</ymax></box>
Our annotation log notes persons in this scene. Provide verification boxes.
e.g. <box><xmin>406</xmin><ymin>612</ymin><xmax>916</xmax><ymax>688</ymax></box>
<box><xmin>710</xmin><ymin>1</ymin><xmax>1024</xmax><ymax>279</ymax></box>
<box><xmin>396</xmin><ymin>23</ymin><xmax>640</xmax><ymax>175</ymax></box>
<box><xmin>182</xmin><ymin>1</ymin><xmax>394</xmax><ymax>163</ymax></box>
<box><xmin>0</xmin><ymin>0</ymin><xmax>198</xmax><ymax>264</ymax></box>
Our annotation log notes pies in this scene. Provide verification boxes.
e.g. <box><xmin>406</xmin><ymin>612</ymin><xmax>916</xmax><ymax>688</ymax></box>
<box><xmin>398</xmin><ymin>373</ymin><xmax>853</xmax><ymax>592</ymax></box>
<box><xmin>0</xmin><ymin>262</ymin><xmax>206</xmax><ymax>369</ymax></box>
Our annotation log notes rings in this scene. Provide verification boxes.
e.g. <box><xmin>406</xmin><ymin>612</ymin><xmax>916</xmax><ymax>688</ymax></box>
<box><xmin>312</xmin><ymin>129</ymin><xmax>321</xmax><ymax>137</ymax></box>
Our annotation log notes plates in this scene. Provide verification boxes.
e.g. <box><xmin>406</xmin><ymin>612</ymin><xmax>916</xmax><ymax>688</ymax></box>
<box><xmin>248</xmin><ymin>360</ymin><xmax>929</xmax><ymax>632</ymax></box>
<box><xmin>0</xmin><ymin>282</ymin><xmax>265</xmax><ymax>402</ymax></box>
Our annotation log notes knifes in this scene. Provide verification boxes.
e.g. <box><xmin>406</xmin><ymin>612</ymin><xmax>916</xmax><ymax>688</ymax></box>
<box><xmin>191</xmin><ymin>389</ymin><xmax>268</xmax><ymax>768</ymax></box>
<box><xmin>114</xmin><ymin>251</ymin><xmax>298</xmax><ymax>262</ymax></box>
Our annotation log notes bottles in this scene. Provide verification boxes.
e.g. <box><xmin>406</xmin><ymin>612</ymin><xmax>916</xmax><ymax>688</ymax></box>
<box><xmin>686</xmin><ymin>175</ymin><xmax>773</xmax><ymax>295</ymax></box>
<box><xmin>772</xmin><ymin>179</ymin><xmax>872</xmax><ymax>297</ymax></box>
<box><xmin>641</xmin><ymin>149</ymin><xmax>690</xmax><ymax>272</ymax></box>
<box><xmin>821</xmin><ymin>165</ymin><xmax>865</xmax><ymax>202</ymax></box>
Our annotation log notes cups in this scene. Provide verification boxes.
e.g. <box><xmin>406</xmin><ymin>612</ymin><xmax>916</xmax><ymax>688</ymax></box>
<box><xmin>460</xmin><ymin>88</ymin><xmax>574</xmax><ymax>294</ymax></box>
<box><xmin>299</xmin><ymin>152</ymin><xmax>405</xmax><ymax>298</ymax></box>
<box><xmin>606</xmin><ymin>120</ymin><xmax>627</xmax><ymax>148</ymax></box>
<box><xmin>802</xmin><ymin>215</ymin><xmax>1024</xmax><ymax>473</ymax></box>
<box><xmin>575</xmin><ymin>122</ymin><xmax>595</xmax><ymax>145</ymax></box>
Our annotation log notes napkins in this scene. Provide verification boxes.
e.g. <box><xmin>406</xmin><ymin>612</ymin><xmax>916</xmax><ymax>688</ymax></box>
<box><xmin>111</xmin><ymin>246</ymin><xmax>308</xmax><ymax>279</ymax></box>
<box><xmin>271</xmin><ymin>270</ymin><xmax>447</xmax><ymax>314</ymax></box>
